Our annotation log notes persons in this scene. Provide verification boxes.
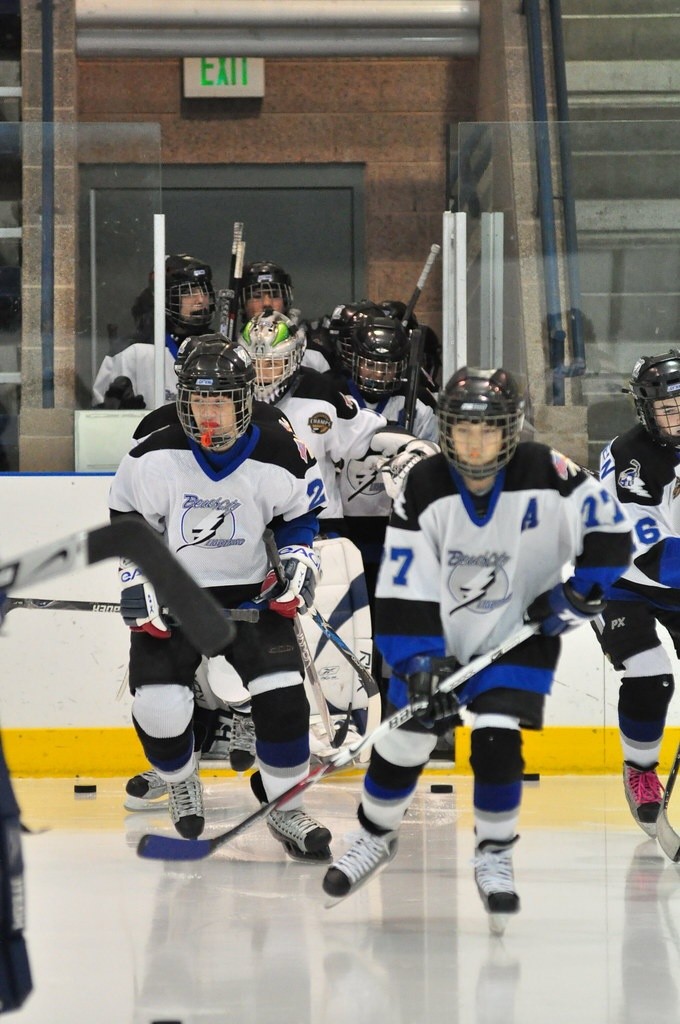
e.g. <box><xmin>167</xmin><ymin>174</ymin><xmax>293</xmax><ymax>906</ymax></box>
<box><xmin>92</xmin><ymin>253</ymin><xmax>442</xmax><ymax>799</ymax></box>
<box><xmin>599</xmin><ymin>347</ymin><xmax>680</xmax><ymax>823</ymax></box>
<box><xmin>321</xmin><ymin>367</ymin><xmax>632</xmax><ymax>916</ymax></box>
<box><xmin>107</xmin><ymin>336</ymin><xmax>332</xmax><ymax>854</ymax></box>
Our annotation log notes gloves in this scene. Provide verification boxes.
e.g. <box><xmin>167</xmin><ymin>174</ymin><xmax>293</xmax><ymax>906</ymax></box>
<box><xmin>118</xmin><ymin>566</ymin><xmax>171</xmax><ymax>640</ymax></box>
<box><xmin>262</xmin><ymin>545</ymin><xmax>324</xmax><ymax>617</ymax></box>
<box><xmin>522</xmin><ymin>580</ymin><xmax>602</xmax><ymax>637</ymax></box>
<box><xmin>407</xmin><ymin>657</ymin><xmax>466</xmax><ymax>728</ymax></box>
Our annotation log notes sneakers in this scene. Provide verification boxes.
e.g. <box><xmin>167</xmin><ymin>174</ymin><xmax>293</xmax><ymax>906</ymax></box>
<box><xmin>250</xmin><ymin>769</ymin><xmax>335</xmax><ymax>864</ymax></box>
<box><xmin>166</xmin><ymin>769</ymin><xmax>206</xmax><ymax>839</ymax></box>
<box><xmin>123</xmin><ymin>768</ymin><xmax>170</xmax><ymax>812</ymax></box>
<box><xmin>229</xmin><ymin>705</ymin><xmax>256</xmax><ymax>771</ymax></box>
<box><xmin>322</xmin><ymin>800</ymin><xmax>408</xmax><ymax>899</ymax></box>
<box><xmin>623</xmin><ymin>759</ymin><xmax>664</xmax><ymax>838</ymax></box>
<box><xmin>473</xmin><ymin>834</ymin><xmax>522</xmax><ymax>914</ymax></box>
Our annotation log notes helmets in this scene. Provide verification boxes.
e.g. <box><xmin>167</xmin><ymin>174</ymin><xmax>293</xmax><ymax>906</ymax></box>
<box><xmin>243</xmin><ymin>262</ymin><xmax>292</xmax><ymax>307</ymax></box>
<box><xmin>438</xmin><ymin>366</ymin><xmax>522</xmax><ymax>480</ymax></box>
<box><xmin>237</xmin><ymin>310</ymin><xmax>308</xmax><ymax>396</ymax></box>
<box><xmin>172</xmin><ymin>332</ymin><xmax>232</xmax><ymax>376</ymax></box>
<box><xmin>178</xmin><ymin>341</ymin><xmax>256</xmax><ymax>447</ymax></box>
<box><xmin>628</xmin><ymin>348</ymin><xmax>680</xmax><ymax>449</ymax></box>
<box><xmin>303</xmin><ymin>298</ymin><xmax>419</xmax><ymax>404</ymax></box>
<box><xmin>148</xmin><ymin>252</ymin><xmax>215</xmax><ymax>333</ymax></box>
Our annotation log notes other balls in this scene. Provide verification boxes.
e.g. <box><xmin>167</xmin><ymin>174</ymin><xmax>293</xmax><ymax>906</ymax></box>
<box><xmin>74</xmin><ymin>785</ymin><xmax>97</xmax><ymax>794</ymax></box>
<box><xmin>431</xmin><ymin>785</ymin><xmax>453</xmax><ymax>793</ymax></box>
<box><xmin>521</xmin><ymin>773</ymin><xmax>539</xmax><ymax>780</ymax></box>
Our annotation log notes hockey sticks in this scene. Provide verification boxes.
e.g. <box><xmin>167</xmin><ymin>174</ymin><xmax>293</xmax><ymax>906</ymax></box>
<box><xmin>229</xmin><ymin>221</ymin><xmax>246</xmax><ymax>289</ymax></box>
<box><xmin>402</xmin><ymin>328</ymin><xmax>423</xmax><ymax>434</ymax></box>
<box><xmin>136</xmin><ymin>623</ymin><xmax>541</xmax><ymax>863</ymax></box>
<box><xmin>590</xmin><ymin>615</ymin><xmax>625</xmax><ymax>678</ymax></box>
<box><xmin>261</xmin><ymin>528</ymin><xmax>355</xmax><ymax>749</ymax></box>
<box><xmin>307</xmin><ymin>602</ymin><xmax>382</xmax><ymax>764</ymax></box>
<box><xmin>0</xmin><ymin>595</ymin><xmax>261</xmax><ymax>629</ymax></box>
<box><xmin>1</xmin><ymin>514</ymin><xmax>237</xmax><ymax>659</ymax></box>
<box><xmin>400</xmin><ymin>243</ymin><xmax>441</xmax><ymax>324</ymax></box>
<box><xmin>655</xmin><ymin>745</ymin><xmax>680</xmax><ymax>864</ymax></box>
<box><xmin>227</xmin><ymin>241</ymin><xmax>246</xmax><ymax>341</ymax></box>
